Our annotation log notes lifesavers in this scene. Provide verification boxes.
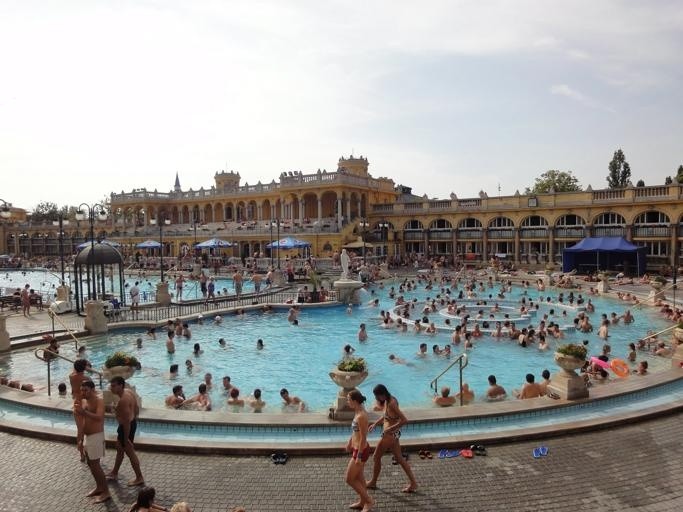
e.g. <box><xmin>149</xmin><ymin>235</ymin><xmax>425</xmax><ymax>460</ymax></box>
<box><xmin>591</xmin><ymin>355</ymin><xmax>611</xmax><ymax>369</ymax></box>
<box><xmin>611</xmin><ymin>359</ymin><xmax>630</xmax><ymax>378</ymax></box>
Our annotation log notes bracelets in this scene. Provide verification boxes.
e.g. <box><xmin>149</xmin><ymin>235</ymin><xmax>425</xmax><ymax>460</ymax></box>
<box><xmin>356</xmin><ymin>450</ymin><xmax>363</xmax><ymax>458</ymax></box>
<box><xmin>384</xmin><ymin>423</ymin><xmax>400</xmax><ymax>432</ymax></box>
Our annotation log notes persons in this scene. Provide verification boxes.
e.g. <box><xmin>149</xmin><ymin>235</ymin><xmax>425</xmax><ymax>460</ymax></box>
<box><xmin>365</xmin><ymin>383</ymin><xmax>418</xmax><ymax>493</ymax></box>
<box><xmin>128</xmin><ymin>486</ymin><xmax>167</xmax><ymax>511</ymax></box>
<box><xmin>169</xmin><ymin>501</ymin><xmax>192</xmax><ymax>512</ymax></box>
<box><xmin>100</xmin><ymin>376</ymin><xmax>144</xmax><ymax>486</ymax></box>
<box><xmin>71</xmin><ymin>381</ymin><xmax>112</xmax><ymax>503</ymax></box>
<box><xmin>0</xmin><ymin>219</ymin><xmax>682</xmax><ymax>412</ymax></box>
<box><xmin>68</xmin><ymin>359</ymin><xmax>91</xmax><ymax>462</ymax></box>
<box><xmin>343</xmin><ymin>388</ymin><xmax>374</xmax><ymax>511</ymax></box>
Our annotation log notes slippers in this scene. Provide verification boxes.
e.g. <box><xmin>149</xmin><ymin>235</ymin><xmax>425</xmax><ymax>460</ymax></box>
<box><xmin>439</xmin><ymin>441</ymin><xmax>486</xmax><ymax>459</ymax></box>
<box><xmin>271</xmin><ymin>454</ymin><xmax>288</xmax><ymax>465</ymax></box>
<box><xmin>419</xmin><ymin>451</ymin><xmax>432</xmax><ymax>459</ymax></box>
<box><xmin>534</xmin><ymin>447</ymin><xmax>547</xmax><ymax>458</ymax></box>
<box><xmin>392</xmin><ymin>455</ymin><xmax>396</xmax><ymax>465</ymax></box>
<box><xmin>403</xmin><ymin>452</ymin><xmax>408</xmax><ymax>462</ymax></box>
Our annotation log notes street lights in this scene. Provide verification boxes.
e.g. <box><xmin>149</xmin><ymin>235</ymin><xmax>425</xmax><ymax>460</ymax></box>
<box><xmin>264</xmin><ymin>222</ymin><xmax>274</xmax><ymax>269</ymax></box>
<box><xmin>149</xmin><ymin>212</ymin><xmax>172</xmax><ymax>283</ymax></box>
<box><xmin>187</xmin><ymin>220</ymin><xmax>202</xmax><ymax>264</ymax></box>
<box><xmin>0</xmin><ymin>199</ymin><xmax>12</xmax><ymax>219</ymax></box>
<box><xmin>358</xmin><ymin>218</ymin><xmax>370</xmax><ymax>267</ymax></box>
<box><xmin>377</xmin><ymin>221</ymin><xmax>388</xmax><ymax>263</ymax></box>
<box><xmin>272</xmin><ymin>217</ymin><xmax>284</xmax><ymax>270</ymax></box>
<box><xmin>75</xmin><ymin>203</ymin><xmax>107</xmax><ymax>301</ymax></box>
<box><xmin>10</xmin><ymin>231</ymin><xmax>65</xmax><ymax>259</ymax></box>
<box><xmin>51</xmin><ymin>211</ymin><xmax>70</xmax><ymax>285</ymax></box>
